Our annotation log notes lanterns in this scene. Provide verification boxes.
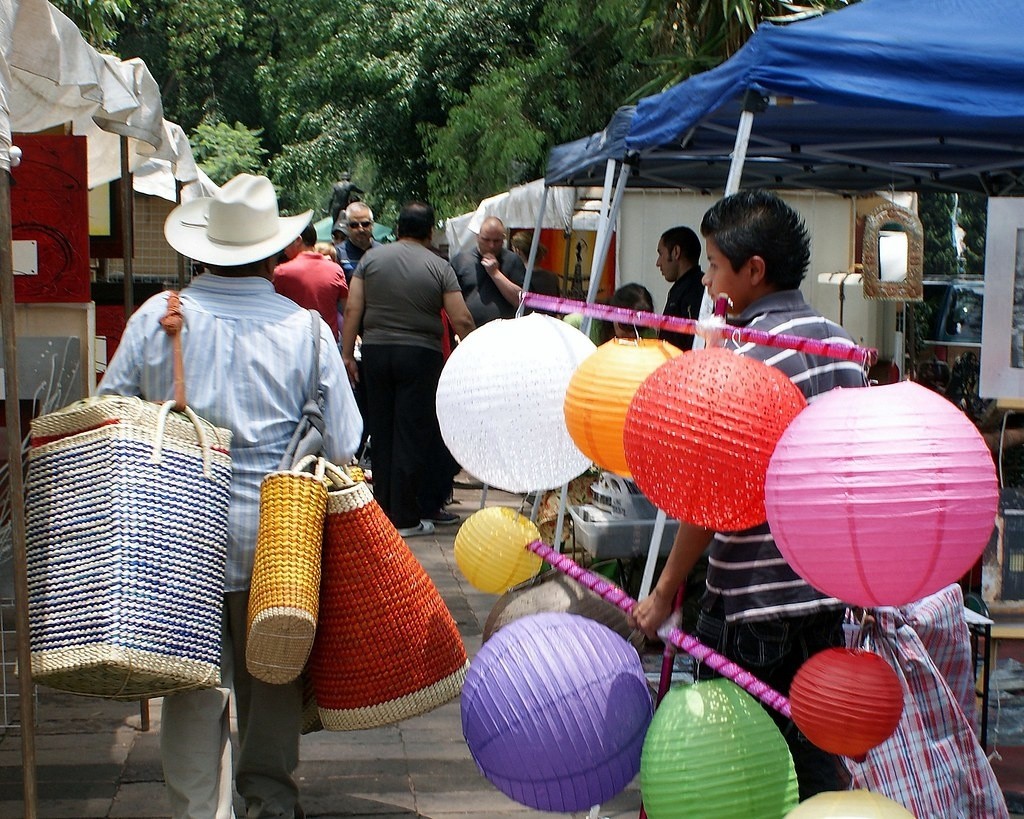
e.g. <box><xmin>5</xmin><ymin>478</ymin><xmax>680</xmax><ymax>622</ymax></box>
<box><xmin>622</xmin><ymin>347</ymin><xmax>807</xmax><ymax>532</ymax></box>
<box><xmin>563</xmin><ymin>336</ymin><xmax>685</xmax><ymax>477</ymax></box>
<box><xmin>782</xmin><ymin>789</ymin><xmax>915</xmax><ymax>819</ymax></box>
<box><xmin>640</xmin><ymin>678</ymin><xmax>799</xmax><ymax>819</ymax></box>
<box><xmin>452</xmin><ymin>510</ymin><xmax>543</xmax><ymax>591</ymax></box>
<box><xmin>789</xmin><ymin>648</ymin><xmax>904</xmax><ymax>757</ymax></box>
<box><xmin>436</xmin><ymin>313</ymin><xmax>596</xmax><ymax>495</ymax></box>
<box><xmin>460</xmin><ymin>613</ymin><xmax>652</xmax><ymax>812</ymax></box>
<box><xmin>764</xmin><ymin>382</ymin><xmax>999</xmax><ymax>606</ymax></box>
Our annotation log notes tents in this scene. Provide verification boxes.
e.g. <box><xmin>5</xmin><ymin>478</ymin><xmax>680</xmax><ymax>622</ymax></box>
<box><xmin>516</xmin><ymin>0</ymin><xmax>1024</xmax><ymax>362</ymax></box>
<box><xmin>0</xmin><ymin>0</ymin><xmax>223</xmax><ymax>819</ymax></box>
<box><xmin>473</xmin><ymin>176</ymin><xmax>618</xmax><ymax>298</ymax></box>
<box><xmin>444</xmin><ymin>212</ymin><xmax>475</xmax><ymax>260</ymax></box>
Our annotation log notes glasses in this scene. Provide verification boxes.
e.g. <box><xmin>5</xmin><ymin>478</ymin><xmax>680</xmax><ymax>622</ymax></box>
<box><xmin>348</xmin><ymin>222</ymin><xmax>371</xmax><ymax>229</ymax></box>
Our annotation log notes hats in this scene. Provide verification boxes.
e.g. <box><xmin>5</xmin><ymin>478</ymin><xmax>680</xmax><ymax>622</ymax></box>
<box><xmin>163</xmin><ymin>173</ymin><xmax>313</xmax><ymax>267</ymax></box>
<box><xmin>331</xmin><ymin>209</ymin><xmax>351</xmax><ymax>238</ymax></box>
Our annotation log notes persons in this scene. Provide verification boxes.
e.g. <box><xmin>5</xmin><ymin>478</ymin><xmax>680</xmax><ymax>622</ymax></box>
<box><xmin>276</xmin><ymin>203</ymin><xmax>475</xmax><ymax>538</ymax></box>
<box><xmin>638</xmin><ymin>192</ymin><xmax>865</xmax><ymax>803</ymax></box>
<box><xmin>513</xmin><ymin>231</ymin><xmax>560</xmax><ymax>318</ymax></box>
<box><xmin>563</xmin><ymin>282</ymin><xmax>659</xmax><ymax>345</ymax></box>
<box><xmin>102</xmin><ymin>174</ymin><xmax>369</xmax><ymax>819</ymax></box>
<box><xmin>657</xmin><ymin>226</ymin><xmax>706</xmax><ymax>350</ymax></box>
<box><xmin>450</xmin><ymin>214</ymin><xmax>526</xmax><ymax>327</ymax></box>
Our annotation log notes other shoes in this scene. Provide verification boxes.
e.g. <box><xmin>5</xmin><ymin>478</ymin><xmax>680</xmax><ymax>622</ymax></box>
<box><xmin>426</xmin><ymin>507</ymin><xmax>461</xmax><ymax>523</ymax></box>
<box><xmin>398</xmin><ymin>520</ymin><xmax>436</xmax><ymax>538</ymax></box>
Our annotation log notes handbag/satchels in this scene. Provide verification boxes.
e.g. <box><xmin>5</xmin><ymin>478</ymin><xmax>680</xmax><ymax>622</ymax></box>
<box><xmin>313</xmin><ymin>459</ymin><xmax>475</xmax><ymax>733</ymax></box>
<box><xmin>246</xmin><ymin>453</ymin><xmax>329</xmax><ymax>686</ymax></box>
<box><xmin>12</xmin><ymin>393</ymin><xmax>235</xmax><ymax>701</ymax></box>
